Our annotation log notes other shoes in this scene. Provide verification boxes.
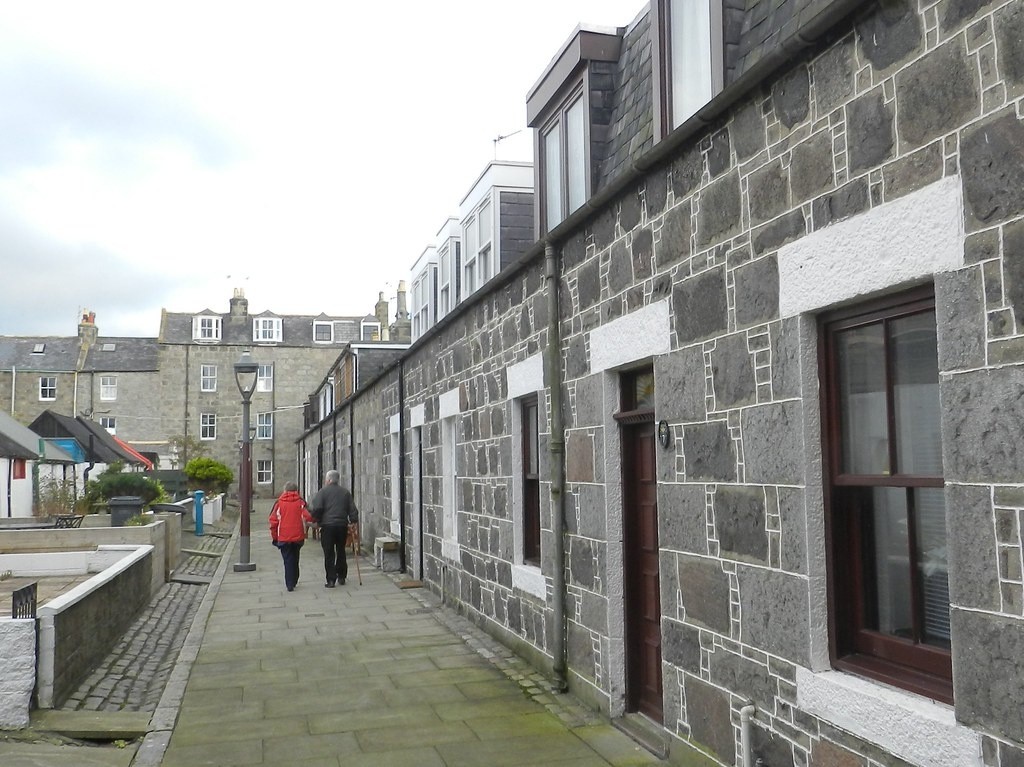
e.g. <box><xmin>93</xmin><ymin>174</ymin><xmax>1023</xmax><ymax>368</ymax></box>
<box><xmin>325</xmin><ymin>582</ymin><xmax>334</xmax><ymax>588</ymax></box>
<box><xmin>337</xmin><ymin>579</ymin><xmax>346</xmax><ymax>585</ymax></box>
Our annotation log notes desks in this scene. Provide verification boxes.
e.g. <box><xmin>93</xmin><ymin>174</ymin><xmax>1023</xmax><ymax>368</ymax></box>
<box><xmin>0</xmin><ymin>523</ymin><xmax>72</xmax><ymax>530</ymax></box>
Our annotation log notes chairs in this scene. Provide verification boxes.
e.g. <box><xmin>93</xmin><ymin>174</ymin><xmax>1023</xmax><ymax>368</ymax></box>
<box><xmin>45</xmin><ymin>514</ymin><xmax>76</xmax><ymax>527</ymax></box>
<box><xmin>54</xmin><ymin>515</ymin><xmax>85</xmax><ymax>529</ymax></box>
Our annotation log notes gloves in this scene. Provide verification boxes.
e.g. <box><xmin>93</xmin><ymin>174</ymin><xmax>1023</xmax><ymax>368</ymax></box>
<box><xmin>272</xmin><ymin>540</ymin><xmax>278</xmax><ymax>546</ymax></box>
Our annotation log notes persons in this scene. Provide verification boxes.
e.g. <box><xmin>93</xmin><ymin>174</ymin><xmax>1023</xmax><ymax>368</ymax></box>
<box><xmin>311</xmin><ymin>470</ymin><xmax>359</xmax><ymax>588</ymax></box>
<box><xmin>268</xmin><ymin>482</ymin><xmax>314</xmax><ymax>592</ymax></box>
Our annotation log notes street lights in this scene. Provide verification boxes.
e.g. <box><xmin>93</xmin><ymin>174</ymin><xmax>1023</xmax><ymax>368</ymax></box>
<box><xmin>248</xmin><ymin>426</ymin><xmax>257</xmax><ymax>510</ymax></box>
<box><xmin>233</xmin><ymin>362</ymin><xmax>259</xmax><ymax>564</ymax></box>
<box><xmin>237</xmin><ymin>439</ymin><xmax>244</xmax><ymax>503</ymax></box>
